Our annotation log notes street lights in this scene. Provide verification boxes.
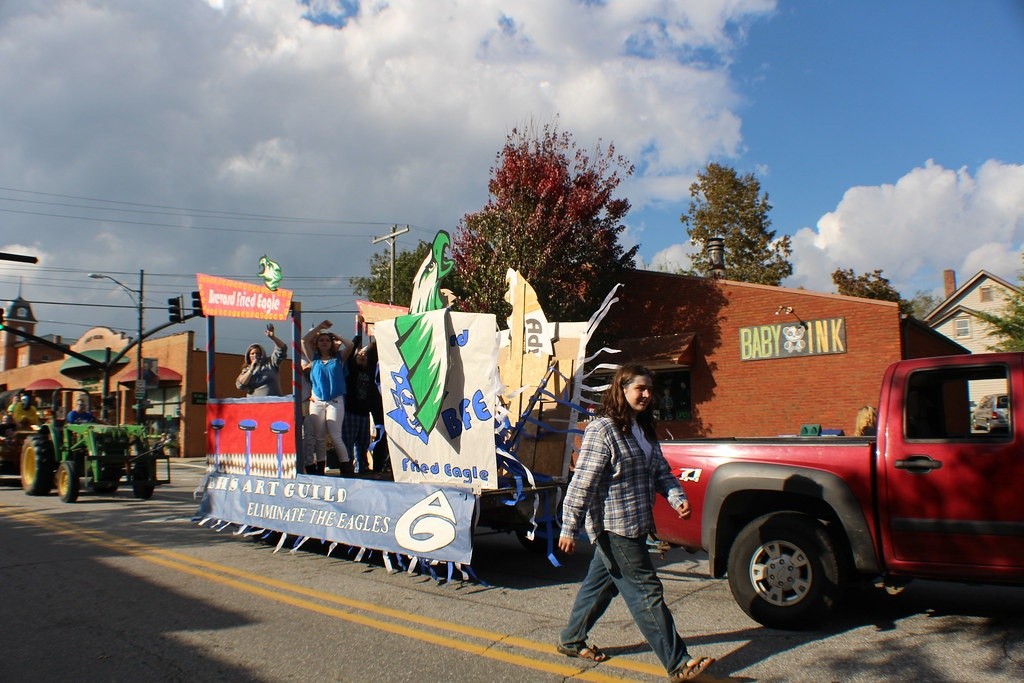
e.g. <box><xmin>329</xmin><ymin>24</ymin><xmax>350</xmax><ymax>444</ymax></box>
<box><xmin>88</xmin><ymin>269</ymin><xmax>144</xmax><ymax>425</ymax></box>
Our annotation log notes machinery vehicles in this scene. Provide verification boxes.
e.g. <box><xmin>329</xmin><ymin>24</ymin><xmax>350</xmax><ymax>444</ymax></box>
<box><xmin>1</xmin><ymin>387</ymin><xmax>171</xmax><ymax>504</ymax></box>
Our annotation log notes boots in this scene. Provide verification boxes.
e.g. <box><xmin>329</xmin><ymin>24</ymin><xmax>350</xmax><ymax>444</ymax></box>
<box><xmin>340</xmin><ymin>462</ymin><xmax>353</xmax><ymax>476</ymax></box>
<box><xmin>305</xmin><ymin>463</ymin><xmax>316</xmax><ymax>477</ymax></box>
<box><xmin>316</xmin><ymin>461</ymin><xmax>328</xmax><ymax>476</ymax></box>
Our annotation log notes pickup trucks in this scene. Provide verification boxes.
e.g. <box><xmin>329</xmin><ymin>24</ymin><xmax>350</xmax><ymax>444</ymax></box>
<box><xmin>646</xmin><ymin>352</ymin><xmax>1024</xmax><ymax>631</ymax></box>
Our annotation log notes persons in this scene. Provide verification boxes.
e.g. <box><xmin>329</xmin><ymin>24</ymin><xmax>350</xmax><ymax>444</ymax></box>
<box><xmin>12</xmin><ymin>393</ymin><xmax>41</xmax><ymax>446</ymax></box>
<box><xmin>370</xmin><ymin>358</ymin><xmax>389</xmax><ymax>481</ymax></box>
<box><xmin>555</xmin><ymin>362</ymin><xmax>717</xmax><ymax>683</ymax></box>
<box><xmin>235</xmin><ymin>322</ymin><xmax>288</xmax><ymax>398</ymax></box>
<box><xmin>7</xmin><ymin>394</ymin><xmax>20</xmax><ymax>416</ymax></box>
<box><xmin>301</xmin><ymin>319</ymin><xmax>355</xmax><ymax>478</ymax></box>
<box><xmin>341</xmin><ymin>335</ymin><xmax>379</xmax><ymax>478</ymax></box>
<box><xmin>299</xmin><ymin>350</ymin><xmax>317</xmax><ymax>476</ymax></box>
<box><xmin>852</xmin><ymin>404</ymin><xmax>878</xmax><ymax>436</ymax></box>
<box><xmin>66</xmin><ymin>398</ymin><xmax>106</xmax><ymax>455</ymax></box>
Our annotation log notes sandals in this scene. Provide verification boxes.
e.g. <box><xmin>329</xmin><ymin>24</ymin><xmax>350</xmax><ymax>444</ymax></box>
<box><xmin>670</xmin><ymin>657</ymin><xmax>713</xmax><ymax>678</ymax></box>
<box><xmin>557</xmin><ymin>640</ymin><xmax>607</xmax><ymax>664</ymax></box>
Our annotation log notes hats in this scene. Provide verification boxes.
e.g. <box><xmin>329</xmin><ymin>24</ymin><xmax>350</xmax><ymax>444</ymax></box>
<box><xmin>311</xmin><ymin>328</ymin><xmax>342</xmax><ymax>352</ymax></box>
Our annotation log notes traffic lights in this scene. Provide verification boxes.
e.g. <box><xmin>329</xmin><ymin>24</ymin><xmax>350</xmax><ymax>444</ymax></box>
<box><xmin>167</xmin><ymin>294</ymin><xmax>186</xmax><ymax>322</ymax></box>
<box><xmin>0</xmin><ymin>307</ymin><xmax>4</xmax><ymax>332</ymax></box>
<box><xmin>191</xmin><ymin>290</ymin><xmax>207</xmax><ymax>318</ymax></box>
